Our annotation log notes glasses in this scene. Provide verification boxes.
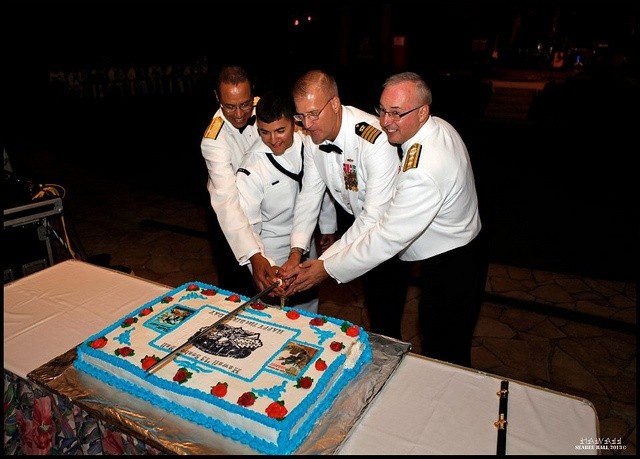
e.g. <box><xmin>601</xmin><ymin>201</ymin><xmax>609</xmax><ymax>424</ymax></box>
<box><xmin>375</xmin><ymin>105</ymin><xmax>422</xmax><ymax>122</ymax></box>
<box><xmin>215</xmin><ymin>97</ymin><xmax>252</xmax><ymax>112</ymax></box>
<box><xmin>293</xmin><ymin>98</ymin><xmax>333</xmax><ymax>122</ymax></box>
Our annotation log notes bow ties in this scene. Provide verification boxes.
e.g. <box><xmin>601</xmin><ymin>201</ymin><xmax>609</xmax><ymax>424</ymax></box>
<box><xmin>319</xmin><ymin>144</ymin><xmax>342</xmax><ymax>155</ymax></box>
<box><xmin>238</xmin><ymin>116</ymin><xmax>255</xmax><ymax>134</ymax></box>
<box><xmin>396</xmin><ymin>143</ymin><xmax>403</xmax><ymax>161</ymax></box>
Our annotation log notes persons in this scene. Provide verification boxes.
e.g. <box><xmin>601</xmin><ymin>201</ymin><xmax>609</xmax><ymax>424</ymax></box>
<box><xmin>235</xmin><ymin>93</ymin><xmax>337</xmax><ymax>314</ymax></box>
<box><xmin>292</xmin><ymin>72</ymin><xmax>489</xmax><ymax>368</ymax></box>
<box><xmin>280</xmin><ymin>69</ymin><xmax>410</xmax><ymax>342</ymax></box>
<box><xmin>200</xmin><ymin>64</ymin><xmax>257</xmax><ymax>299</ymax></box>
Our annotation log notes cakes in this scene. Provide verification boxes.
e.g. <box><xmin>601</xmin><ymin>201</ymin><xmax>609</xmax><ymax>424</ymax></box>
<box><xmin>71</xmin><ymin>280</ymin><xmax>372</xmax><ymax>455</ymax></box>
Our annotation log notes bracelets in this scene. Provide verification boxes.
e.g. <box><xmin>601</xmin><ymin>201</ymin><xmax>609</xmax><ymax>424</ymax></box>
<box><xmin>288</xmin><ymin>248</ymin><xmax>302</xmax><ymax>257</ymax></box>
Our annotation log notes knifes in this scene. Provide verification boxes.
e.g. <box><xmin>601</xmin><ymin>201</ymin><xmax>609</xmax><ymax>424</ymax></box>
<box><xmin>146</xmin><ymin>276</ymin><xmax>286</xmax><ymax>376</ymax></box>
